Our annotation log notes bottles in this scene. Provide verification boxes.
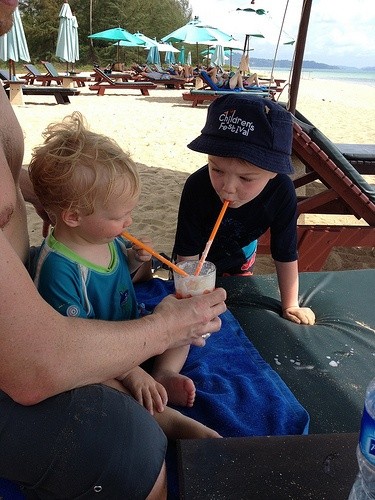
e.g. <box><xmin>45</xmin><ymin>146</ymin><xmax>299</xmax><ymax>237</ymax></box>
<box><xmin>347</xmin><ymin>376</ymin><xmax>375</xmax><ymax>500</ymax></box>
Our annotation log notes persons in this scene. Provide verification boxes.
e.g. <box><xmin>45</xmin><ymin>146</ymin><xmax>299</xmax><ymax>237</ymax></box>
<box><xmin>25</xmin><ymin>110</ymin><xmax>225</xmax><ymax>439</ymax></box>
<box><xmin>206</xmin><ymin>68</ymin><xmax>247</xmax><ymax>92</ymax></box>
<box><xmin>133</xmin><ymin>61</ymin><xmax>244</xmax><ymax>85</ymax></box>
<box><xmin>168</xmin><ymin>95</ymin><xmax>317</xmax><ymax>326</ymax></box>
<box><xmin>0</xmin><ymin>1</ymin><xmax>226</xmax><ymax>500</ymax></box>
<box><xmin>241</xmin><ymin>74</ymin><xmax>262</xmax><ymax>89</ymax></box>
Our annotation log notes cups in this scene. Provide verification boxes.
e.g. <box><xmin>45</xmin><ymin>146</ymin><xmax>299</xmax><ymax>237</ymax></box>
<box><xmin>172</xmin><ymin>259</ymin><xmax>216</xmax><ymax>340</ymax></box>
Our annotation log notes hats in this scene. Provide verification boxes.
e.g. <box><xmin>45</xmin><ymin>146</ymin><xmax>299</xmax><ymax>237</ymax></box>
<box><xmin>186</xmin><ymin>92</ymin><xmax>297</xmax><ymax>175</ymax></box>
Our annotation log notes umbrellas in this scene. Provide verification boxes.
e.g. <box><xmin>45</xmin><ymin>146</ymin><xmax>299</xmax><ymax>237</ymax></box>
<box><xmin>53</xmin><ymin>1</ymin><xmax>80</xmax><ymax>76</ymax></box>
<box><xmin>86</xmin><ymin>15</ymin><xmax>267</xmax><ymax>75</ymax></box>
<box><xmin>0</xmin><ymin>8</ymin><xmax>31</xmax><ymax>80</ymax></box>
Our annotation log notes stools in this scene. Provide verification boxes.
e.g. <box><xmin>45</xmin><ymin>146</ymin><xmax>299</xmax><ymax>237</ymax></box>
<box><xmin>131</xmin><ymin>269</ymin><xmax>375</xmax><ymax>437</ymax></box>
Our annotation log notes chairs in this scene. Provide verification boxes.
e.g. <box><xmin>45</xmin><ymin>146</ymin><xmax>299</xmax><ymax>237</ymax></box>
<box><xmin>0</xmin><ymin>60</ymin><xmax>274</xmax><ymax>108</ymax></box>
<box><xmin>275</xmin><ymin>101</ymin><xmax>375</xmax><ymax>272</ymax></box>
